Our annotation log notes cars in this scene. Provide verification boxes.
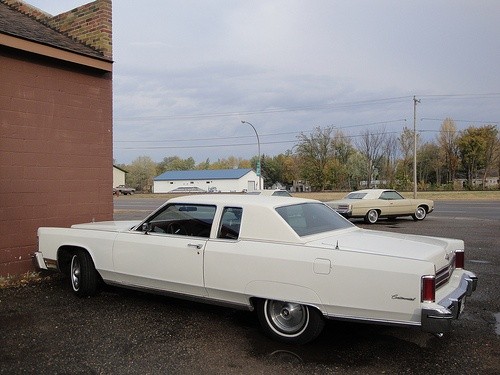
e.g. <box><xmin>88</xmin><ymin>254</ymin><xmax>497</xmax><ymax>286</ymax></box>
<box><xmin>30</xmin><ymin>193</ymin><xmax>478</xmax><ymax>348</ymax></box>
<box><xmin>324</xmin><ymin>189</ymin><xmax>434</xmax><ymax>224</ymax></box>
<box><xmin>248</xmin><ymin>191</ymin><xmax>291</xmax><ymax>198</ymax></box>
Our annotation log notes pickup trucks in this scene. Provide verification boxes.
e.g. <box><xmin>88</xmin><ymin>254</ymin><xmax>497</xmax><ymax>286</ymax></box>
<box><xmin>112</xmin><ymin>185</ymin><xmax>136</xmax><ymax>195</ymax></box>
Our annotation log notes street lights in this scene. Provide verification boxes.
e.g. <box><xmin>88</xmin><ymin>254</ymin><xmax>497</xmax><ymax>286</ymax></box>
<box><xmin>241</xmin><ymin>121</ymin><xmax>261</xmax><ymax>189</ymax></box>
